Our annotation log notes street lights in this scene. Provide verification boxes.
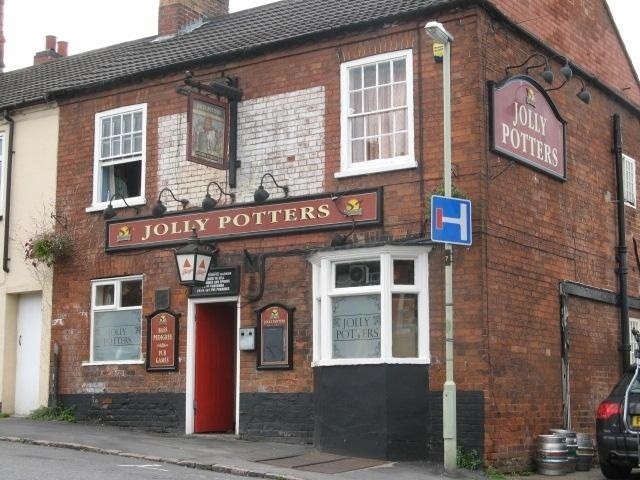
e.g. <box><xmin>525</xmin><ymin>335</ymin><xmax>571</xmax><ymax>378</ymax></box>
<box><xmin>422</xmin><ymin>21</ymin><xmax>460</xmax><ymax>475</ymax></box>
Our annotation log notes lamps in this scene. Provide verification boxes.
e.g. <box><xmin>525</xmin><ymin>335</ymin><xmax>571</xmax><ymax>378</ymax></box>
<box><xmin>254</xmin><ymin>173</ymin><xmax>288</xmax><ymax>205</ymax></box>
<box><xmin>332</xmin><ymin>192</ymin><xmax>356</xmax><ymax>246</ymax></box>
<box><xmin>173</xmin><ymin>228</ymin><xmax>219</xmax><ymax>287</ymax></box>
<box><xmin>152</xmin><ymin>188</ymin><xmax>188</xmax><ymax>218</ymax></box>
<box><xmin>104</xmin><ymin>194</ymin><xmax>138</xmax><ymax>220</ymax></box>
<box><xmin>505</xmin><ymin>53</ymin><xmax>592</xmax><ymax>104</ymax></box>
<box><xmin>202</xmin><ymin>182</ymin><xmax>235</xmax><ymax>212</ymax></box>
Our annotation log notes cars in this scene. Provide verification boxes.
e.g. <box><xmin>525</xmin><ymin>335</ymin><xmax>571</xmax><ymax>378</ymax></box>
<box><xmin>595</xmin><ymin>363</ymin><xmax>640</xmax><ymax>480</ymax></box>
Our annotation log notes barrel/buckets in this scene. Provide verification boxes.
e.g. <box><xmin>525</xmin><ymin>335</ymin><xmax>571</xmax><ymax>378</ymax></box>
<box><xmin>535</xmin><ymin>429</ymin><xmax>595</xmax><ymax>477</ymax></box>
